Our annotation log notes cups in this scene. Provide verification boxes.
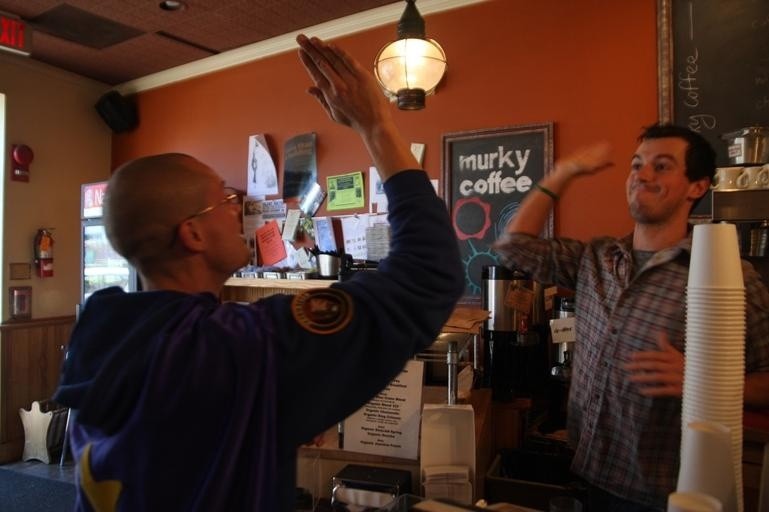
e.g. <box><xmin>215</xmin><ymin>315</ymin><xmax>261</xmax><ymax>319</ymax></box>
<box><xmin>670</xmin><ymin>223</ymin><xmax>750</xmax><ymax>512</ymax></box>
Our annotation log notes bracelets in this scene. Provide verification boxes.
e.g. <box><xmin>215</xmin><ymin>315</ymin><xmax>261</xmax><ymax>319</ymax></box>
<box><xmin>535</xmin><ymin>183</ymin><xmax>561</xmax><ymax>203</ymax></box>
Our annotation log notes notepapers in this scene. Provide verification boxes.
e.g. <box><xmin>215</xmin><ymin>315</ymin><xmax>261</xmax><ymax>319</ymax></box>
<box><xmin>317</xmin><ymin>221</ymin><xmax>338</xmax><ymax>253</ymax></box>
<box><xmin>281</xmin><ymin>209</ymin><xmax>301</xmax><ymax>243</ymax></box>
<box><xmin>313</xmin><ymin>217</ymin><xmax>327</xmax><ymax>247</ymax></box>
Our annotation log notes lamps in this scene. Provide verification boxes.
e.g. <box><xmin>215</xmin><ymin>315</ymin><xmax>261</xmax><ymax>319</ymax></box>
<box><xmin>374</xmin><ymin>0</ymin><xmax>447</xmax><ymax>110</ymax></box>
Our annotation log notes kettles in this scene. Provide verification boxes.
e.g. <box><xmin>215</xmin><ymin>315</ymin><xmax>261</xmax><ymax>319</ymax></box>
<box><xmin>308</xmin><ymin>246</ymin><xmax>353</xmax><ymax>280</ymax></box>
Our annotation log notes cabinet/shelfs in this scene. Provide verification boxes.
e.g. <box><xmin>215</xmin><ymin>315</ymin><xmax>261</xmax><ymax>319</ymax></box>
<box><xmin>224</xmin><ymin>278</ymin><xmax>339</xmax><ymax>288</ymax></box>
<box><xmin>297</xmin><ymin>332</ymin><xmax>482</xmax><ymax>505</ymax></box>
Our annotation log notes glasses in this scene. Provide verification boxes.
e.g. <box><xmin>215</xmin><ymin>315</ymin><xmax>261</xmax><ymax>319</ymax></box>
<box><xmin>188</xmin><ymin>187</ymin><xmax>242</xmax><ymax>220</ymax></box>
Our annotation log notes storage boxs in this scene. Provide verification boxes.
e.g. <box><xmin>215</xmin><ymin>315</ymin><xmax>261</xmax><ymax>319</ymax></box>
<box><xmin>486</xmin><ymin>448</ymin><xmax>590</xmax><ymax>512</ymax></box>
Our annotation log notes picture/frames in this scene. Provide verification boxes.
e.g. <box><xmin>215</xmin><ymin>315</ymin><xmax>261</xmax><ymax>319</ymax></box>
<box><xmin>439</xmin><ymin>120</ymin><xmax>554</xmax><ymax>308</ymax></box>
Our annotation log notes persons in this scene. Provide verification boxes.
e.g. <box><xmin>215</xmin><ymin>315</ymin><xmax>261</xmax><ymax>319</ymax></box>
<box><xmin>49</xmin><ymin>31</ymin><xmax>466</xmax><ymax>512</ymax></box>
<box><xmin>490</xmin><ymin>124</ymin><xmax>768</xmax><ymax>512</ymax></box>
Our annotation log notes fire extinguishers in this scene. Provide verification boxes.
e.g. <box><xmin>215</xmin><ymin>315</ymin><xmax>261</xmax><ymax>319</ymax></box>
<box><xmin>33</xmin><ymin>227</ymin><xmax>55</xmax><ymax>278</ymax></box>
